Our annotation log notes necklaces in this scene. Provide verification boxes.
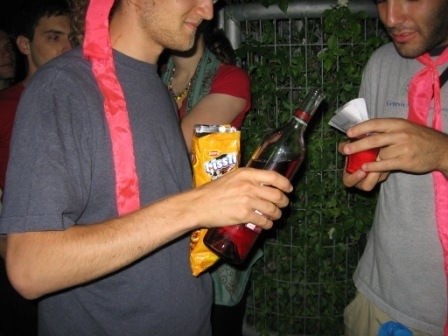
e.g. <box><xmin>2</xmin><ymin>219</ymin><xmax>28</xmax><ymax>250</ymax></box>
<box><xmin>168</xmin><ymin>67</ymin><xmax>191</xmax><ymax>101</ymax></box>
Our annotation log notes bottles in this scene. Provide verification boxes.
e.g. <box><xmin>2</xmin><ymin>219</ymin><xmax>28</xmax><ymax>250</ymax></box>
<box><xmin>202</xmin><ymin>87</ymin><xmax>327</xmax><ymax>272</ymax></box>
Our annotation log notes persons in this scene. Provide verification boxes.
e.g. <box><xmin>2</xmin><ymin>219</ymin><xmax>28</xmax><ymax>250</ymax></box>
<box><xmin>339</xmin><ymin>0</ymin><xmax>448</xmax><ymax>336</ymax></box>
<box><xmin>0</xmin><ymin>0</ymin><xmax>293</xmax><ymax>336</ymax></box>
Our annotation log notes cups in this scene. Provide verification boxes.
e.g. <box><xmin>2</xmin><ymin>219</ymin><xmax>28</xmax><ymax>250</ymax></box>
<box><xmin>346</xmin><ymin>133</ymin><xmax>381</xmax><ymax>176</ymax></box>
